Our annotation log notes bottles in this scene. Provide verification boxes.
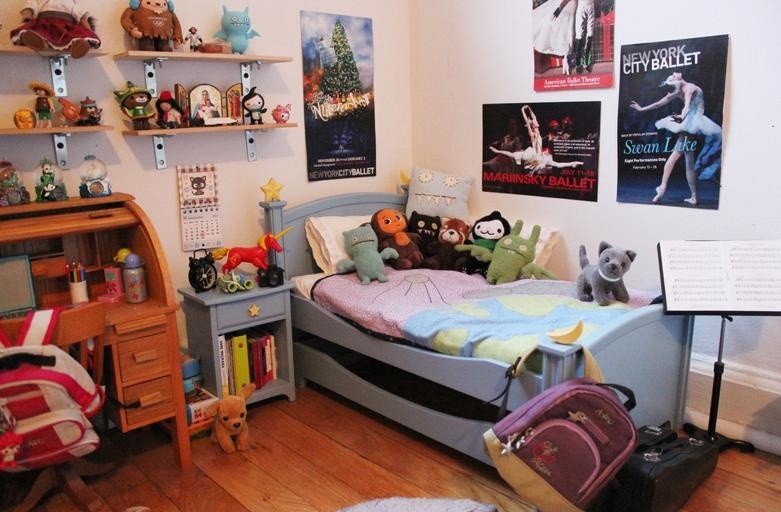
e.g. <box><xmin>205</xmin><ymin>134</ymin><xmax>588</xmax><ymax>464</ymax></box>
<box><xmin>123</xmin><ymin>254</ymin><xmax>148</xmax><ymax>303</ymax></box>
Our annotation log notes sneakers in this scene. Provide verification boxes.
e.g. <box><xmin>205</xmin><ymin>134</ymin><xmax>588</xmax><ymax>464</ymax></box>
<box><xmin>571</xmin><ymin>162</ymin><xmax>584</xmax><ymax>168</ymax></box>
<box><xmin>653</xmin><ymin>187</ymin><xmax>662</xmax><ymax>202</ymax></box>
<box><xmin>489</xmin><ymin>146</ymin><xmax>497</xmax><ymax>153</ymax></box>
<box><xmin>683</xmin><ymin>197</ymin><xmax>696</xmax><ymax>205</ymax></box>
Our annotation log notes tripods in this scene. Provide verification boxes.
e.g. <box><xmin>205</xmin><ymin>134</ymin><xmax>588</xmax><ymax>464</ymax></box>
<box><xmin>683</xmin><ymin>316</ymin><xmax>756</xmax><ymax>459</ymax></box>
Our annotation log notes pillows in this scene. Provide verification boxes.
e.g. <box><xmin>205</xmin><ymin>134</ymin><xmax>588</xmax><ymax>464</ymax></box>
<box><xmin>403</xmin><ymin>167</ymin><xmax>476</xmax><ymax>222</ymax></box>
<box><xmin>304</xmin><ymin>215</ymin><xmax>375</xmax><ymax>275</ymax></box>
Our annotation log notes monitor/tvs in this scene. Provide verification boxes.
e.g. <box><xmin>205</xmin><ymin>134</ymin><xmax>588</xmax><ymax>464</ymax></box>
<box><xmin>0</xmin><ymin>255</ymin><xmax>38</xmax><ymax>320</ymax></box>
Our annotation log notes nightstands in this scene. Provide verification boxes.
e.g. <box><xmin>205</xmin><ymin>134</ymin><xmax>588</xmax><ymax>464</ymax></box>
<box><xmin>178</xmin><ymin>275</ymin><xmax>297</xmax><ymax>407</ymax></box>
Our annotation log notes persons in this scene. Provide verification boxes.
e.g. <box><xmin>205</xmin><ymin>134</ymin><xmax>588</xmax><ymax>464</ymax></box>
<box><xmin>630</xmin><ymin>72</ymin><xmax>722</xmax><ymax>205</ymax></box>
<box><xmin>192</xmin><ymin>103</ymin><xmax>207</xmax><ymax>127</ymax></box>
<box><xmin>191</xmin><ymin>90</ymin><xmax>220</xmax><ymax>120</ymax></box>
<box><xmin>203</xmin><ymin>99</ymin><xmax>219</xmax><ymax>118</ymax></box>
<box><xmin>483</xmin><ymin>104</ymin><xmax>601</xmax><ymax>176</ymax></box>
<box><xmin>569</xmin><ymin>1</ymin><xmax>602</xmax><ymax>74</ymax></box>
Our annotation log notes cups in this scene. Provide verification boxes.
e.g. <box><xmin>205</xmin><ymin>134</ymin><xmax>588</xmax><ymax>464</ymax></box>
<box><xmin>69</xmin><ymin>280</ymin><xmax>90</xmax><ymax>304</ymax></box>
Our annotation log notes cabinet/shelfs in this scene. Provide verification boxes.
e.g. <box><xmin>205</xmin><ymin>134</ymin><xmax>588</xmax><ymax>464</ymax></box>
<box><xmin>113</xmin><ymin>50</ymin><xmax>299</xmax><ymax>171</ymax></box>
<box><xmin>1</xmin><ymin>192</ymin><xmax>193</xmax><ymax>469</ymax></box>
<box><xmin>0</xmin><ymin>46</ymin><xmax>116</xmax><ymax>172</ymax></box>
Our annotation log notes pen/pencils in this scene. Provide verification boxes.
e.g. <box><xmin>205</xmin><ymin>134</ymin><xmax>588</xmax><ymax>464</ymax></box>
<box><xmin>66</xmin><ymin>262</ymin><xmax>87</xmax><ymax>282</ymax></box>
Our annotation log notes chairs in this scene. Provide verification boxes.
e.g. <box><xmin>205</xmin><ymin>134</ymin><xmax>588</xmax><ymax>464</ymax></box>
<box><xmin>2</xmin><ymin>301</ymin><xmax>113</xmax><ymax>512</ymax></box>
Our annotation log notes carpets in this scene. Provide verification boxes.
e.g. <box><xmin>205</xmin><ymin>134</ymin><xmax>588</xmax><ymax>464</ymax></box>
<box><xmin>336</xmin><ymin>497</ymin><xmax>497</xmax><ymax>512</ymax></box>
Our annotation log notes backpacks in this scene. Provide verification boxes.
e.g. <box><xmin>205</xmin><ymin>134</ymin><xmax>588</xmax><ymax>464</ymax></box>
<box><xmin>599</xmin><ymin>421</ymin><xmax>719</xmax><ymax>512</ymax></box>
<box><xmin>0</xmin><ymin>308</ymin><xmax>106</xmax><ymax>472</ymax></box>
<box><xmin>483</xmin><ymin>342</ymin><xmax>638</xmax><ymax>512</ymax></box>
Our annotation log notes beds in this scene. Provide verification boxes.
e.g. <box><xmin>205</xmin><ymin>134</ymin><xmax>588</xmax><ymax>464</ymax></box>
<box><xmin>260</xmin><ymin>170</ymin><xmax>700</xmax><ymax>481</ymax></box>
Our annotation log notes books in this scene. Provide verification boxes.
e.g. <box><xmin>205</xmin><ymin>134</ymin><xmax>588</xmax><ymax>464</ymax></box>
<box><xmin>217</xmin><ymin>328</ymin><xmax>280</xmax><ymax>397</ymax></box>
<box><xmin>658</xmin><ymin>239</ymin><xmax>781</xmax><ymax>315</ymax></box>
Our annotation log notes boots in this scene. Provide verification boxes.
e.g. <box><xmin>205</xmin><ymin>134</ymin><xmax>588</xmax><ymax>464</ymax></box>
<box><xmin>583</xmin><ymin>37</ymin><xmax>593</xmax><ymax>72</ymax></box>
<box><xmin>573</xmin><ymin>37</ymin><xmax>583</xmax><ymax>74</ymax></box>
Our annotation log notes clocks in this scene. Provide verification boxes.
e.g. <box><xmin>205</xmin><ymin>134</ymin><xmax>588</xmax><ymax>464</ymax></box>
<box><xmin>188</xmin><ymin>249</ymin><xmax>217</xmax><ymax>293</ymax></box>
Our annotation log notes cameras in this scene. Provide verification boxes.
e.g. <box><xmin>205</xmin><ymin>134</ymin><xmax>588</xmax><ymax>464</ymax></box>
<box><xmin>256</xmin><ymin>262</ymin><xmax>286</xmax><ymax>289</ymax></box>
